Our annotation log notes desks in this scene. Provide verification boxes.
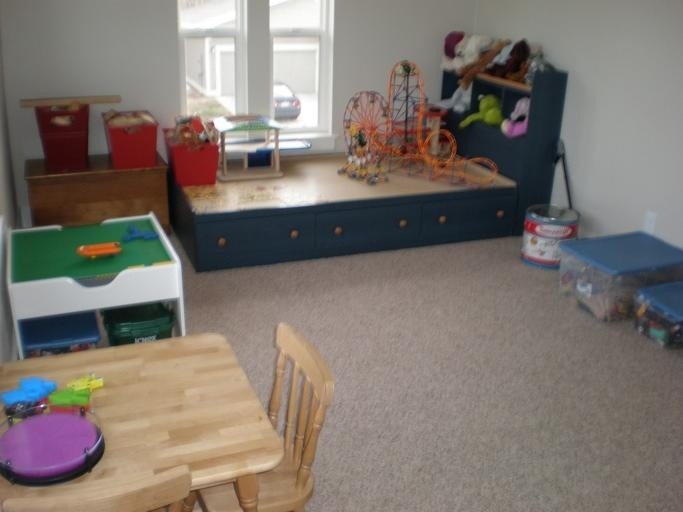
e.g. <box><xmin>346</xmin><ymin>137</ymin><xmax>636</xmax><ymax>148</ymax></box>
<box><xmin>0</xmin><ymin>331</ymin><xmax>286</xmax><ymax>512</ymax></box>
<box><xmin>5</xmin><ymin>211</ymin><xmax>185</xmax><ymax>360</ymax></box>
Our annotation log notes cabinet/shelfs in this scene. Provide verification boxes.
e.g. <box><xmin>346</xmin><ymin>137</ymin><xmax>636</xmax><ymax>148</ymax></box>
<box><xmin>22</xmin><ymin>150</ymin><xmax>174</xmax><ymax>236</ymax></box>
<box><xmin>193</xmin><ymin>193</ymin><xmax>520</xmax><ymax>272</ymax></box>
<box><xmin>443</xmin><ymin>64</ymin><xmax>534</xmax><ymax>144</ymax></box>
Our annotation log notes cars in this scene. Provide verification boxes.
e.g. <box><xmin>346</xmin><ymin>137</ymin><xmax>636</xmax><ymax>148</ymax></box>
<box><xmin>273</xmin><ymin>83</ymin><xmax>301</xmax><ymax>119</ymax></box>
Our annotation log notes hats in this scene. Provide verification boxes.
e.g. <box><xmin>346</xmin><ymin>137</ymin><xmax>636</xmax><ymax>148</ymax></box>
<box><xmin>444</xmin><ymin>31</ymin><xmax>464</xmax><ymax>58</ymax></box>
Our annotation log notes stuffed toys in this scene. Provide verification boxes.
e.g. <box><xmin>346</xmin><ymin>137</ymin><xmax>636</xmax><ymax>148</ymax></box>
<box><xmin>439</xmin><ymin>31</ymin><xmax>547</xmax><ymax>140</ymax></box>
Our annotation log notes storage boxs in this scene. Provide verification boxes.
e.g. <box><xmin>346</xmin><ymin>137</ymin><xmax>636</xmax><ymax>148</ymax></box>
<box><xmin>16</xmin><ymin>303</ymin><xmax>176</xmax><ymax>359</ymax></box>
<box><xmin>556</xmin><ymin>229</ymin><xmax>682</xmax><ymax>359</ymax></box>
<box><xmin>35</xmin><ymin>103</ymin><xmax>219</xmax><ymax>187</ymax></box>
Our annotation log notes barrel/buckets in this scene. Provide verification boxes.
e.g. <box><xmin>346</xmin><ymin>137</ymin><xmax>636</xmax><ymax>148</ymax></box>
<box><xmin>521</xmin><ymin>202</ymin><xmax>578</xmax><ymax>271</ymax></box>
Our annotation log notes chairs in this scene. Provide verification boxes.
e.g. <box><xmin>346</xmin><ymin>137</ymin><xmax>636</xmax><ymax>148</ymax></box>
<box><xmin>0</xmin><ymin>465</ymin><xmax>191</xmax><ymax>512</ymax></box>
<box><xmin>185</xmin><ymin>323</ymin><xmax>332</xmax><ymax>512</ymax></box>
